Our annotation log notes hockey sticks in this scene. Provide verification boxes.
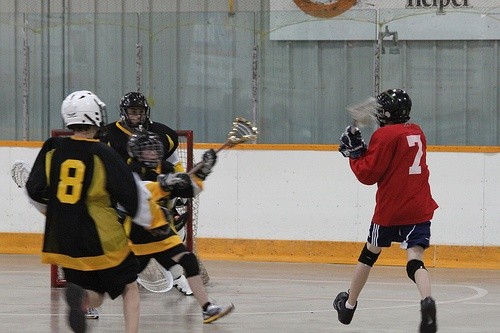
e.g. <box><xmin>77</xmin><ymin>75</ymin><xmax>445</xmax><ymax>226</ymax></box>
<box><xmin>11</xmin><ymin>160</ymin><xmax>32</xmax><ymax>187</ymax></box>
<box><xmin>187</xmin><ymin>116</ymin><xmax>258</xmax><ymax>175</ymax></box>
<box><xmin>345</xmin><ymin>97</ymin><xmax>386</xmax><ymax>130</ymax></box>
<box><xmin>135</xmin><ymin>258</ymin><xmax>174</xmax><ymax>293</ymax></box>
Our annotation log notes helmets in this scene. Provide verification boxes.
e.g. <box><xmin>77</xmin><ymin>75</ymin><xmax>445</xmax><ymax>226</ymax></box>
<box><xmin>120</xmin><ymin>92</ymin><xmax>151</xmax><ymax>124</ymax></box>
<box><xmin>377</xmin><ymin>88</ymin><xmax>413</xmax><ymax>124</ymax></box>
<box><xmin>128</xmin><ymin>131</ymin><xmax>164</xmax><ymax>165</ymax></box>
<box><xmin>60</xmin><ymin>90</ymin><xmax>107</xmax><ymax>128</ymax></box>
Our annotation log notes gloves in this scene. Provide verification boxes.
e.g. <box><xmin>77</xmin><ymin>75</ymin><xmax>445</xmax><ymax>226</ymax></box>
<box><xmin>157</xmin><ymin>172</ymin><xmax>189</xmax><ymax>194</ymax></box>
<box><xmin>338</xmin><ymin>125</ymin><xmax>367</xmax><ymax>158</ymax></box>
<box><xmin>203</xmin><ymin>148</ymin><xmax>218</xmax><ymax>169</ymax></box>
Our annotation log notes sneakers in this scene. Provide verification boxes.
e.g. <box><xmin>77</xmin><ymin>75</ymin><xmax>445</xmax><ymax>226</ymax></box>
<box><xmin>202</xmin><ymin>302</ymin><xmax>234</xmax><ymax>323</ymax></box>
<box><xmin>173</xmin><ymin>275</ymin><xmax>193</xmax><ymax>295</ymax></box>
<box><xmin>333</xmin><ymin>291</ymin><xmax>358</xmax><ymax>325</ymax></box>
<box><xmin>65</xmin><ymin>286</ymin><xmax>86</xmax><ymax>333</ymax></box>
<box><xmin>417</xmin><ymin>295</ymin><xmax>437</xmax><ymax>333</ymax></box>
<box><xmin>84</xmin><ymin>307</ymin><xmax>99</xmax><ymax>319</ymax></box>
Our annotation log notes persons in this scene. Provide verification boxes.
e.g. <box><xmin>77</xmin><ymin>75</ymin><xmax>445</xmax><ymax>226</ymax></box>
<box><xmin>83</xmin><ymin>132</ymin><xmax>234</xmax><ymax>323</ymax></box>
<box><xmin>26</xmin><ymin>90</ymin><xmax>152</xmax><ymax>333</ymax></box>
<box><xmin>107</xmin><ymin>91</ymin><xmax>196</xmax><ymax>296</ymax></box>
<box><xmin>333</xmin><ymin>88</ymin><xmax>438</xmax><ymax>333</ymax></box>
